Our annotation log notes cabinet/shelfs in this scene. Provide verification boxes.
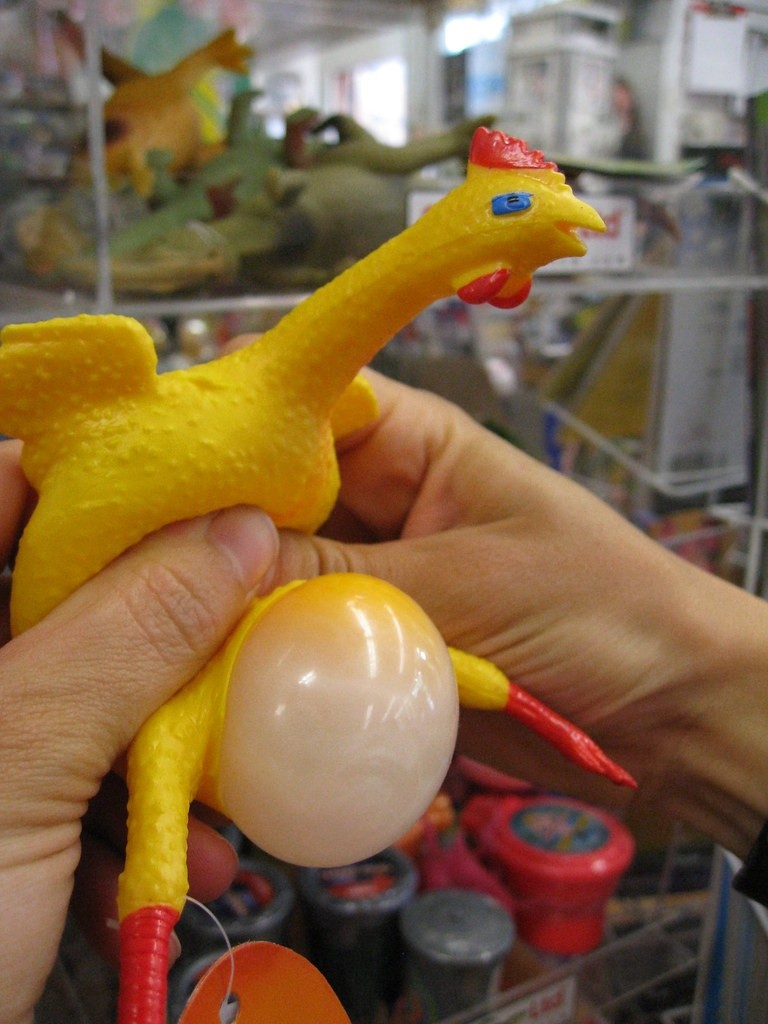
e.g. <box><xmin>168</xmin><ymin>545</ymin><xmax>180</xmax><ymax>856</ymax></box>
<box><xmin>0</xmin><ymin>0</ymin><xmax>767</xmax><ymax>598</ymax></box>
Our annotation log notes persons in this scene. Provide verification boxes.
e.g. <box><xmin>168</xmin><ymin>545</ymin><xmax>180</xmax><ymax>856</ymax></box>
<box><xmin>0</xmin><ymin>332</ymin><xmax>768</xmax><ymax>1024</ymax></box>
